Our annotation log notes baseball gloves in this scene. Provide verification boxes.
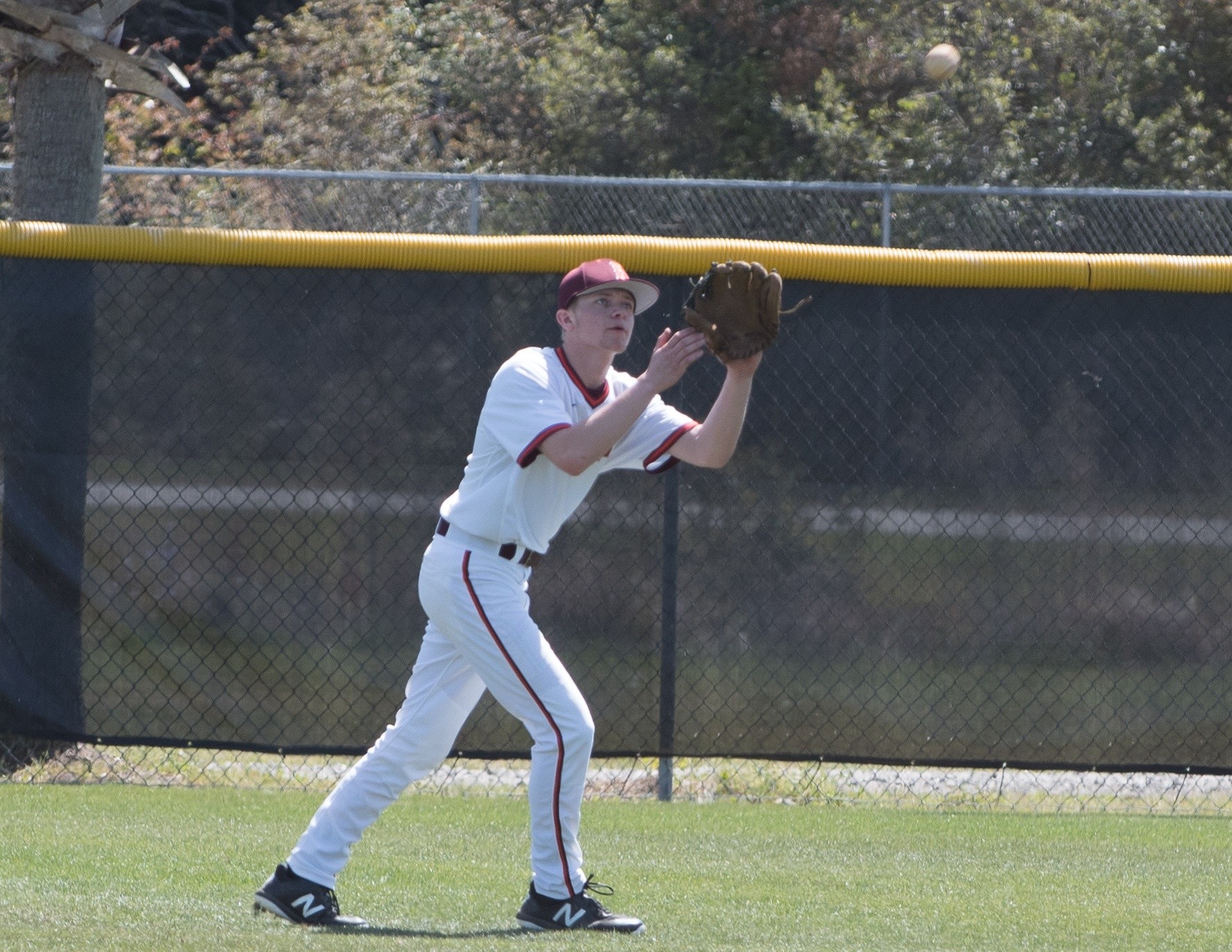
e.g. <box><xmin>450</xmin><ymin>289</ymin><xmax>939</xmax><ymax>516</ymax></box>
<box><xmin>681</xmin><ymin>258</ymin><xmax>784</xmax><ymax>365</ymax></box>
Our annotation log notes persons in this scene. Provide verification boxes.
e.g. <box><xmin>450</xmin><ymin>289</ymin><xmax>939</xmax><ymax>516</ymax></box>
<box><xmin>255</xmin><ymin>256</ymin><xmax>783</xmax><ymax>936</ymax></box>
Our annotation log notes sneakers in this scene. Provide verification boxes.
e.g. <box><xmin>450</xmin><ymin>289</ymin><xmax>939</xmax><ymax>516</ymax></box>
<box><xmin>516</xmin><ymin>873</ymin><xmax>647</xmax><ymax>935</ymax></box>
<box><xmin>254</xmin><ymin>863</ymin><xmax>368</xmax><ymax>928</ymax></box>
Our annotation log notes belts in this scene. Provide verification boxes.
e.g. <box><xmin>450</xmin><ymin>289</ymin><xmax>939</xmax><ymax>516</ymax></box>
<box><xmin>436</xmin><ymin>518</ymin><xmax>543</xmax><ymax>568</ymax></box>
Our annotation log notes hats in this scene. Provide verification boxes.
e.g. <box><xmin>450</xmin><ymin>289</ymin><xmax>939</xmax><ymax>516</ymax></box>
<box><xmin>558</xmin><ymin>256</ymin><xmax>659</xmax><ymax>315</ymax></box>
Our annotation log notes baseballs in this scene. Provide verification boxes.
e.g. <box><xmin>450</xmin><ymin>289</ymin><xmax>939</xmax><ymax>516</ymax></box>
<box><xmin>923</xmin><ymin>43</ymin><xmax>962</xmax><ymax>83</ymax></box>
